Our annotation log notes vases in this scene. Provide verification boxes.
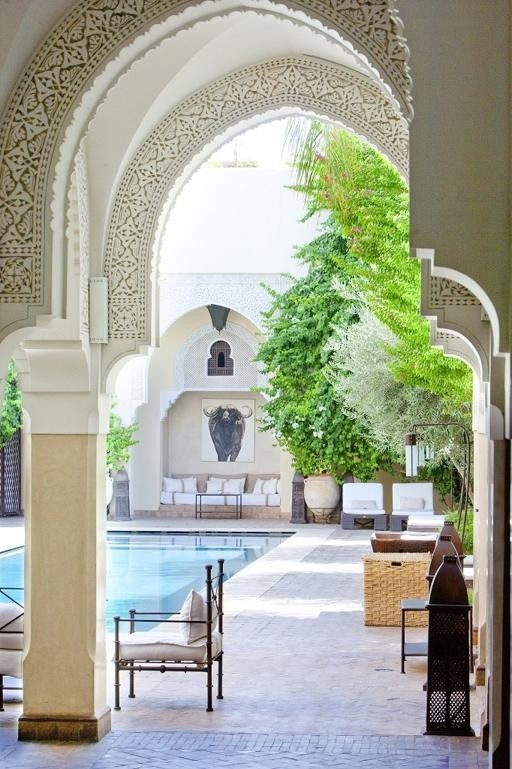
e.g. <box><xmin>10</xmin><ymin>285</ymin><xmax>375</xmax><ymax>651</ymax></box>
<box><xmin>302</xmin><ymin>473</ymin><xmax>342</xmax><ymax>525</ymax></box>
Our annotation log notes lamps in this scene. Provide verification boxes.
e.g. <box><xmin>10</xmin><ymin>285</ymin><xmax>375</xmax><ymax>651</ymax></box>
<box><xmin>424</xmin><ymin>519</ymin><xmax>477</xmax><ymax>740</ymax></box>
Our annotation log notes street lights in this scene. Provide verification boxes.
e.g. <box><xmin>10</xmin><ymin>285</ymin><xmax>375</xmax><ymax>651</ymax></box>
<box><xmin>404</xmin><ymin>422</ymin><xmax>471</xmax><ymax>544</ymax></box>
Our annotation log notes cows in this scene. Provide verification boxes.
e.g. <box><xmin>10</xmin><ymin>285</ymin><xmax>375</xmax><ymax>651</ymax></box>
<box><xmin>202</xmin><ymin>403</ymin><xmax>254</xmax><ymax>463</ymax></box>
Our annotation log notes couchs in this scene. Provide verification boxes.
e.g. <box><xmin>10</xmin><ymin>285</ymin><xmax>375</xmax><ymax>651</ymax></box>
<box><xmin>162</xmin><ymin>472</ymin><xmax>281</xmax><ymax>507</ymax></box>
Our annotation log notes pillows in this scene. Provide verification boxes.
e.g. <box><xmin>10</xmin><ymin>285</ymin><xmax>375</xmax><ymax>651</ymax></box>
<box><xmin>180</xmin><ymin>589</ymin><xmax>218</xmax><ymax>643</ymax></box>
<box><xmin>165</xmin><ymin>476</ymin><xmax>198</xmax><ymax>493</ymax></box>
<box><xmin>205</xmin><ymin>477</ymin><xmax>246</xmax><ymax>495</ymax></box>
<box><xmin>253</xmin><ymin>477</ymin><xmax>280</xmax><ymax>494</ymax></box>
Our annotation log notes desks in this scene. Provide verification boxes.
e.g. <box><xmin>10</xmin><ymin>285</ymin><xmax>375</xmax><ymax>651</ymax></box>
<box><xmin>400</xmin><ymin>599</ymin><xmax>473</xmax><ymax>676</ymax></box>
<box><xmin>371</xmin><ymin>529</ymin><xmax>436</xmax><ymax>552</ymax></box>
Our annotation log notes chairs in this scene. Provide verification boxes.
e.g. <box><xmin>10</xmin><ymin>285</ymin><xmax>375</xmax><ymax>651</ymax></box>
<box><xmin>112</xmin><ymin>559</ymin><xmax>226</xmax><ymax>714</ymax></box>
<box><xmin>1</xmin><ymin>587</ymin><xmax>29</xmax><ymax>712</ymax></box>
<box><xmin>340</xmin><ymin>482</ymin><xmax>447</xmax><ymax>530</ymax></box>
